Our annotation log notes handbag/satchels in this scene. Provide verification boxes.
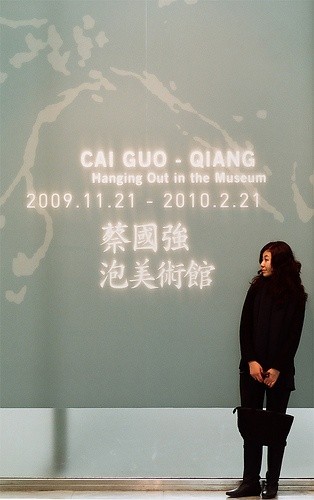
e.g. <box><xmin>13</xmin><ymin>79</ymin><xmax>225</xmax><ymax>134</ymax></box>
<box><xmin>232</xmin><ymin>372</ymin><xmax>295</xmax><ymax>445</ymax></box>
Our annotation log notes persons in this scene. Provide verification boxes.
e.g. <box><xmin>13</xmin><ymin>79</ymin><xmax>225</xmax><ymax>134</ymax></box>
<box><xmin>224</xmin><ymin>240</ymin><xmax>308</xmax><ymax>499</ymax></box>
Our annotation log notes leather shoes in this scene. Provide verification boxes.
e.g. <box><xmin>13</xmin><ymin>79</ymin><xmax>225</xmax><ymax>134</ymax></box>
<box><xmin>226</xmin><ymin>479</ymin><xmax>261</xmax><ymax>497</ymax></box>
<box><xmin>262</xmin><ymin>483</ymin><xmax>279</xmax><ymax>499</ymax></box>
